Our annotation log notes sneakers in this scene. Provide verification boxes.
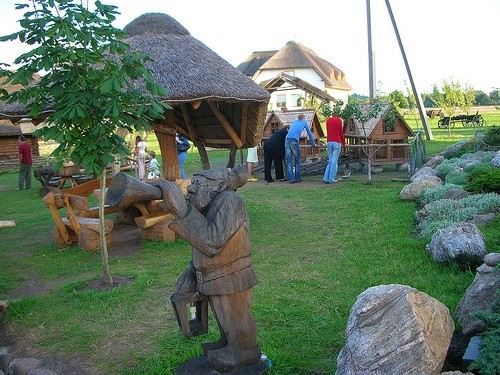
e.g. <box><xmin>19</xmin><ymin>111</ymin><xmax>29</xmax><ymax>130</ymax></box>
<box><xmin>248</xmin><ymin>177</ymin><xmax>258</xmax><ymax>182</ymax></box>
<box><xmin>330</xmin><ymin>180</ymin><xmax>337</xmax><ymax>183</ymax></box>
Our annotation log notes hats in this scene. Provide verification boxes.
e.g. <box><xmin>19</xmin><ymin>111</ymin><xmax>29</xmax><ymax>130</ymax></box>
<box><xmin>18</xmin><ymin>135</ymin><xmax>26</xmax><ymax>141</ymax></box>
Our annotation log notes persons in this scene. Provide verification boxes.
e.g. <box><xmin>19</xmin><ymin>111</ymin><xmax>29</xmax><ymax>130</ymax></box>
<box><xmin>263</xmin><ymin>125</ymin><xmax>290</xmax><ymax>183</ymax></box>
<box><xmin>16</xmin><ymin>135</ymin><xmax>33</xmax><ymax>191</ymax></box>
<box><xmin>153</xmin><ymin>164</ymin><xmax>263</xmax><ymax>372</ymax></box>
<box><xmin>147</xmin><ymin>151</ymin><xmax>161</xmax><ymax>179</ymax></box>
<box><xmin>322</xmin><ymin>112</ymin><xmax>348</xmax><ymax>184</ymax></box>
<box><xmin>247</xmin><ymin>145</ymin><xmax>259</xmax><ymax>182</ymax></box>
<box><xmin>135</xmin><ymin>135</ymin><xmax>147</xmax><ymax>181</ymax></box>
<box><xmin>175</xmin><ymin>132</ymin><xmax>191</xmax><ymax>180</ymax></box>
<box><xmin>284</xmin><ymin>114</ymin><xmax>318</xmax><ymax>184</ymax></box>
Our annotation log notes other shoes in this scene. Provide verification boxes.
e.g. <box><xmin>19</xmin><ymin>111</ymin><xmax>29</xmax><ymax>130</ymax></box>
<box><xmin>268</xmin><ymin>180</ymin><xmax>275</xmax><ymax>182</ymax></box>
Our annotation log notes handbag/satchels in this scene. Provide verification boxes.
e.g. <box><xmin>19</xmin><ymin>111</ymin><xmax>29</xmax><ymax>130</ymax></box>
<box><xmin>177</xmin><ymin>137</ymin><xmax>191</xmax><ymax>151</ymax></box>
